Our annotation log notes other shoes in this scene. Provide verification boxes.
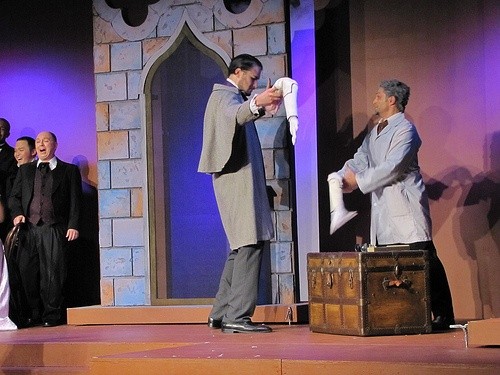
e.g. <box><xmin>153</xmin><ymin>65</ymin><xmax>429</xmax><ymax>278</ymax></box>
<box><xmin>42</xmin><ymin>318</ymin><xmax>60</xmax><ymax>327</ymax></box>
<box><xmin>431</xmin><ymin>314</ymin><xmax>452</xmax><ymax>330</ymax></box>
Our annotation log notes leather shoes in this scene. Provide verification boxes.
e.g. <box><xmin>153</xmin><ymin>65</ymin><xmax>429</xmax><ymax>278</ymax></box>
<box><xmin>221</xmin><ymin>319</ymin><xmax>271</xmax><ymax>333</ymax></box>
<box><xmin>207</xmin><ymin>317</ymin><xmax>221</xmax><ymax>329</ymax></box>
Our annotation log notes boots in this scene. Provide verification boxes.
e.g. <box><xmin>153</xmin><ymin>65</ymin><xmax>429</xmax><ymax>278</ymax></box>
<box><xmin>327</xmin><ymin>171</ymin><xmax>360</xmax><ymax>237</ymax></box>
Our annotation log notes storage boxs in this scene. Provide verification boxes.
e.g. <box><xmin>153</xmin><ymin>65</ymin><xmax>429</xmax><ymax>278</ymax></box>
<box><xmin>468</xmin><ymin>318</ymin><xmax>500</xmax><ymax>348</ymax></box>
<box><xmin>307</xmin><ymin>252</ymin><xmax>431</xmax><ymax>337</ymax></box>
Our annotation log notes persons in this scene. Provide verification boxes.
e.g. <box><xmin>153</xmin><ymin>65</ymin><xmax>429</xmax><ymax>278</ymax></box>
<box><xmin>197</xmin><ymin>54</ymin><xmax>283</xmax><ymax>333</ymax></box>
<box><xmin>0</xmin><ymin>117</ymin><xmax>84</xmax><ymax>330</ymax></box>
<box><xmin>335</xmin><ymin>79</ymin><xmax>456</xmax><ymax>330</ymax></box>
